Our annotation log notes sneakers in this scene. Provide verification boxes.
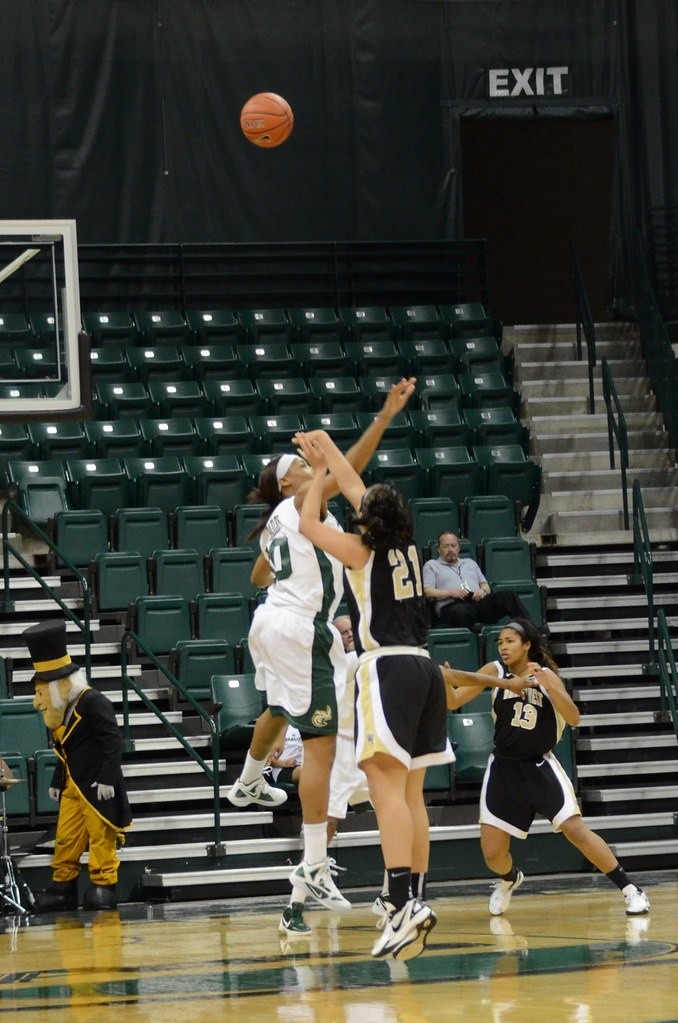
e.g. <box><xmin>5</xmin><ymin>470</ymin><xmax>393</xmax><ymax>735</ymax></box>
<box><xmin>621</xmin><ymin>884</ymin><xmax>651</xmax><ymax>915</ymax></box>
<box><xmin>627</xmin><ymin>917</ymin><xmax>651</xmax><ymax>947</ymax></box>
<box><xmin>393</xmin><ymin>902</ymin><xmax>437</xmax><ymax>961</ymax></box>
<box><xmin>489</xmin><ymin>867</ymin><xmax>524</xmax><ymax>916</ymax></box>
<box><xmin>289</xmin><ymin>856</ymin><xmax>352</xmax><ymax>913</ymax></box>
<box><xmin>491</xmin><ymin>918</ymin><xmax>519</xmax><ymax>954</ymax></box>
<box><xmin>279</xmin><ymin>901</ymin><xmax>312</xmax><ymax>936</ymax></box>
<box><xmin>371</xmin><ymin>897</ymin><xmax>431</xmax><ymax>957</ymax></box>
<box><xmin>227</xmin><ymin>766</ymin><xmax>287</xmax><ymax>807</ymax></box>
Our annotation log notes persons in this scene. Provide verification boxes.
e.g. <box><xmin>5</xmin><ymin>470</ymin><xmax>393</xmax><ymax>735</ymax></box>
<box><xmin>227</xmin><ymin>376</ymin><xmax>540</xmax><ymax>958</ymax></box>
<box><xmin>444</xmin><ymin>619</ymin><xmax>650</xmax><ymax>916</ymax></box>
<box><xmin>422</xmin><ymin>531</ymin><xmax>547</xmax><ymax>640</ymax></box>
<box><xmin>22</xmin><ymin>618</ymin><xmax>133</xmax><ymax>913</ymax></box>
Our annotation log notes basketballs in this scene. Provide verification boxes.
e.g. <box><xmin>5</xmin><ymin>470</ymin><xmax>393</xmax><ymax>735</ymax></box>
<box><xmin>239</xmin><ymin>91</ymin><xmax>295</xmax><ymax>149</ymax></box>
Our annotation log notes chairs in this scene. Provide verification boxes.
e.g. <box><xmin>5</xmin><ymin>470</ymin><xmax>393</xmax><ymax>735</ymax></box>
<box><xmin>0</xmin><ymin>302</ymin><xmax>576</xmax><ymax>828</ymax></box>
